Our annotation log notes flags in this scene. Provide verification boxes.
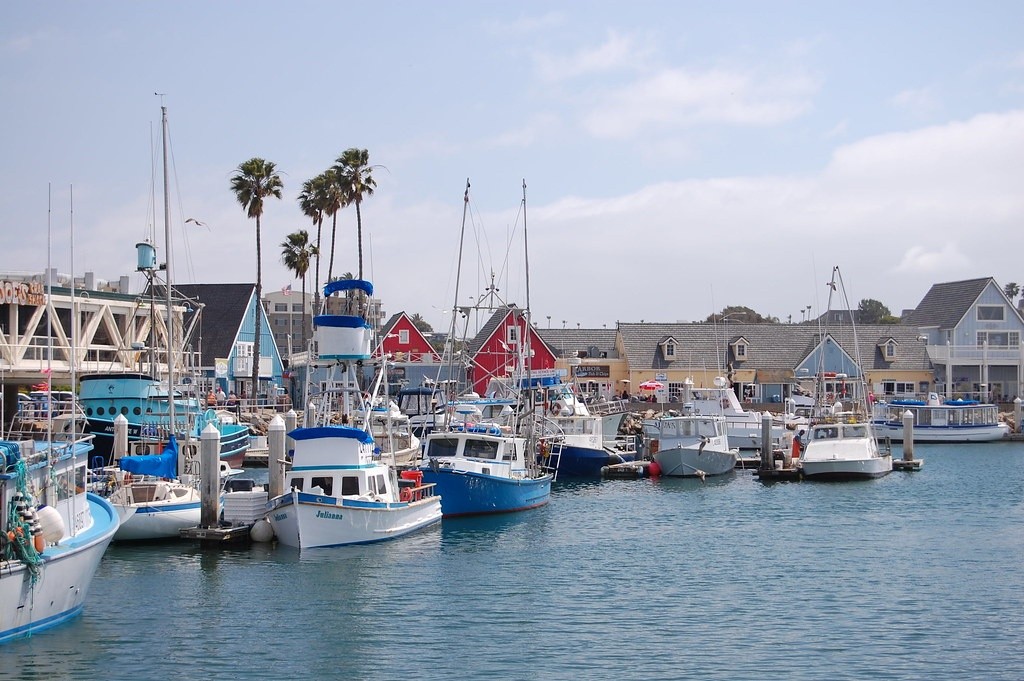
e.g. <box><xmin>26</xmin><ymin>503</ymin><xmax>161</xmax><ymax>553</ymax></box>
<box><xmin>282</xmin><ymin>284</ymin><xmax>292</xmax><ymax>295</ymax></box>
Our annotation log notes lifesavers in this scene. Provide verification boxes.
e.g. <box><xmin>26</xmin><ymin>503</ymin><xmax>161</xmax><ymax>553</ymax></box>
<box><xmin>551</xmin><ymin>403</ymin><xmax>562</xmax><ymax>417</ymax></box>
<box><xmin>400</xmin><ymin>486</ymin><xmax>412</xmax><ymax>501</ymax></box>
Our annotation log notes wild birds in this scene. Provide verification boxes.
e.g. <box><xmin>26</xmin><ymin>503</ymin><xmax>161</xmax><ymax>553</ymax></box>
<box><xmin>184</xmin><ymin>217</ymin><xmax>212</xmax><ymax>232</ymax></box>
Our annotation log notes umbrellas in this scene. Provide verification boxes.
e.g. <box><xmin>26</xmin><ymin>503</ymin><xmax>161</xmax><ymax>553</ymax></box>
<box><xmin>639</xmin><ymin>379</ymin><xmax>664</xmax><ymax>402</ymax></box>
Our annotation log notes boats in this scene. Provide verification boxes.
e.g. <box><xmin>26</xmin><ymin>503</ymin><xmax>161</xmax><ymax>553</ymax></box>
<box><xmin>793</xmin><ymin>264</ymin><xmax>895</xmax><ymax>480</ymax></box>
<box><xmin>863</xmin><ymin>392</ymin><xmax>1011</xmax><ymax>444</ymax></box>
<box><xmin>51</xmin><ymin>240</ymin><xmax>844</xmax><ymax>541</ymax></box>
<box><xmin>404</xmin><ymin>176</ymin><xmax>569</xmax><ymax>517</ymax></box>
<box><xmin>0</xmin><ymin>182</ymin><xmax>120</xmax><ymax>647</ymax></box>
<box><xmin>256</xmin><ymin>295</ymin><xmax>444</xmax><ymax>553</ymax></box>
<box><xmin>649</xmin><ymin>317</ymin><xmax>739</xmax><ymax>478</ymax></box>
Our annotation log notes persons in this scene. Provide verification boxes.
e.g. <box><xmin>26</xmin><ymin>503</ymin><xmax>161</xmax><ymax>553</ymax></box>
<box><xmin>338</xmin><ymin>393</ymin><xmax>343</xmax><ymax>405</ymax></box>
<box><xmin>643</xmin><ymin>393</ymin><xmax>657</xmax><ymax>403</ymax></box>
<box><xmin>586</xmin><ymin>394</ymin><xmax>592</xmax><ymax>405</ymax></box>
<box><xmin>792</xmin><ymin>430</ymin><xmax>807</xmax><ymax>458</ymax></box>
<box><xmin>208</xmin><ymin>388</ymin><xmax>237</xmax><ymax>412</ymax></box>
<box><xmin>869</xmin><ymin>391</ymin><xmax>874</xmax><ymax>403</ymax></box>
<box><xmin>364</xmin><ymin>389</ymin><xmax>370</xmax><ymax>406</ymax></box>
<box><xmin>450</xmin><ymin>391</ymin><xmax>456</xmax><ymax>401</ymax></box>
<box><xmin>613</xmin><ymin>390</ymin><xmax>629</xmax><ymax>401</ymax></box>
<box><xmin>598</xmin><ymin>395</ymin><xmax>606</xmax><ymax>404</ymax></box>
<box><xmin>578</xmin><ymin>394</ymin><xmax>584</xmax><ymax>403</ymax></box>
<box><xmin>799</xmin><ymin>392</ymin><xmax>814</xmax><ymax>407</ymax></box>
<box><xmin>827</xmin><ymin>393</ymin><xmax>835</xmax><ymax>406</ymax></box>
<box><xmin>696</xmin><ymin>392</ymin><xmax>701</xmax><ymax>399</ymax></box>
<box><xmin>350</xmin><ymin>392</ymin><xmax>356</xmax><ymax>410</ymax></box>
<box><xmin>241</xmin><ymin>390</ymin><xmax>248</xmax><ymax>412</ymax></box>
